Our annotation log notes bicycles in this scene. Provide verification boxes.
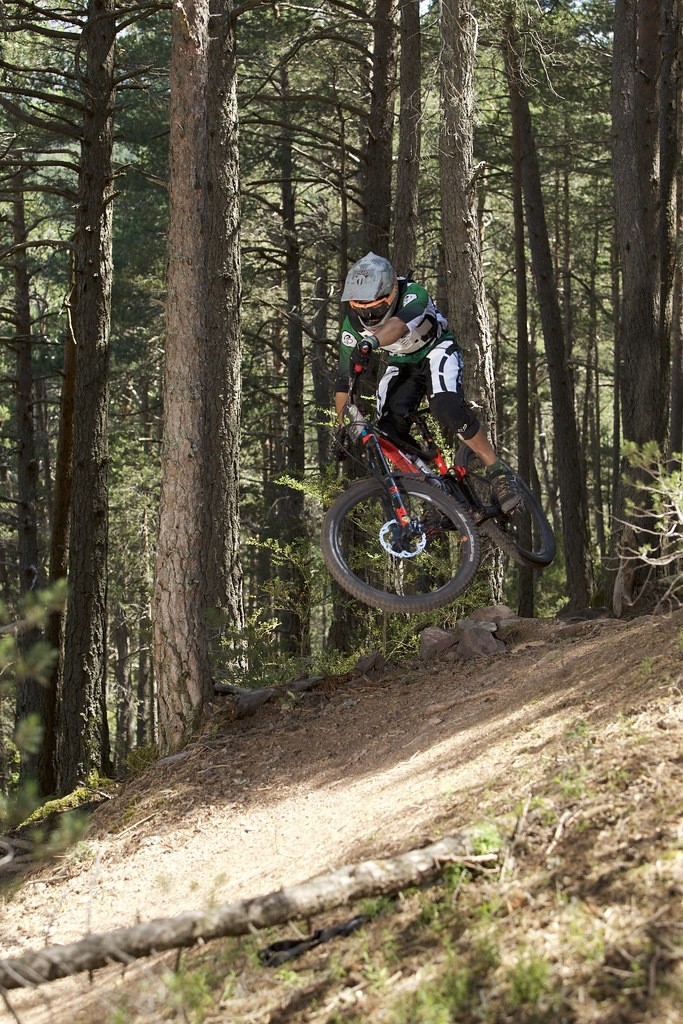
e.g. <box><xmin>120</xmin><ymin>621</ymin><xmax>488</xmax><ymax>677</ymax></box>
<box><xmin>320</xmin><ymin>342</ymin><xmax>558</xmax><ymax>613</ymax></box>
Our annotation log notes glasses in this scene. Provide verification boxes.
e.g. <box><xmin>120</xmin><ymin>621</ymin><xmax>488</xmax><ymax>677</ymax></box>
<box><xmin>349</xmin><ymin>295</ymin><xmax>392</xmax><ymax>322</ymax></box>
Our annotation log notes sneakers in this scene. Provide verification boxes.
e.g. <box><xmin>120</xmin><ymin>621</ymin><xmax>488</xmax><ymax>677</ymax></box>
<box><xmin>487</xmin><ymin>465</ymin><xmax>526</xmax><ymax>514</ymax></box>
<box><xmin>422</xmin><ymin>501</ymin><xmax>458</xmax><ymax>531</ymax></box>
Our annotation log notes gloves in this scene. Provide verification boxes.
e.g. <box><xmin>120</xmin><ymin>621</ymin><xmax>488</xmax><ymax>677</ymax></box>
<box><xmin>331</xmin><ymin>423</ymin><xmax>352</xmax><ymax>460</ymax></box>
<box><xmin>349</xmin><ymin>335</ymin><xmax>379</xmax><ymax>379</ymax></box>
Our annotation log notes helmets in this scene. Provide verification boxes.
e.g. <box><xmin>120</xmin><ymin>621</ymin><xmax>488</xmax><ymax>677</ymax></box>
<box><xmin>340</xmin><ymin>252</ymin><xmax>398</xmax><ymax>334</ymax></box>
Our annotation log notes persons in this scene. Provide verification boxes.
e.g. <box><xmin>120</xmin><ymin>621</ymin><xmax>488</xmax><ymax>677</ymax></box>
<box><xmin>335</xmin><ymin>252</ymin><xmax>526</xmax><ymax>516</ymax></box>
